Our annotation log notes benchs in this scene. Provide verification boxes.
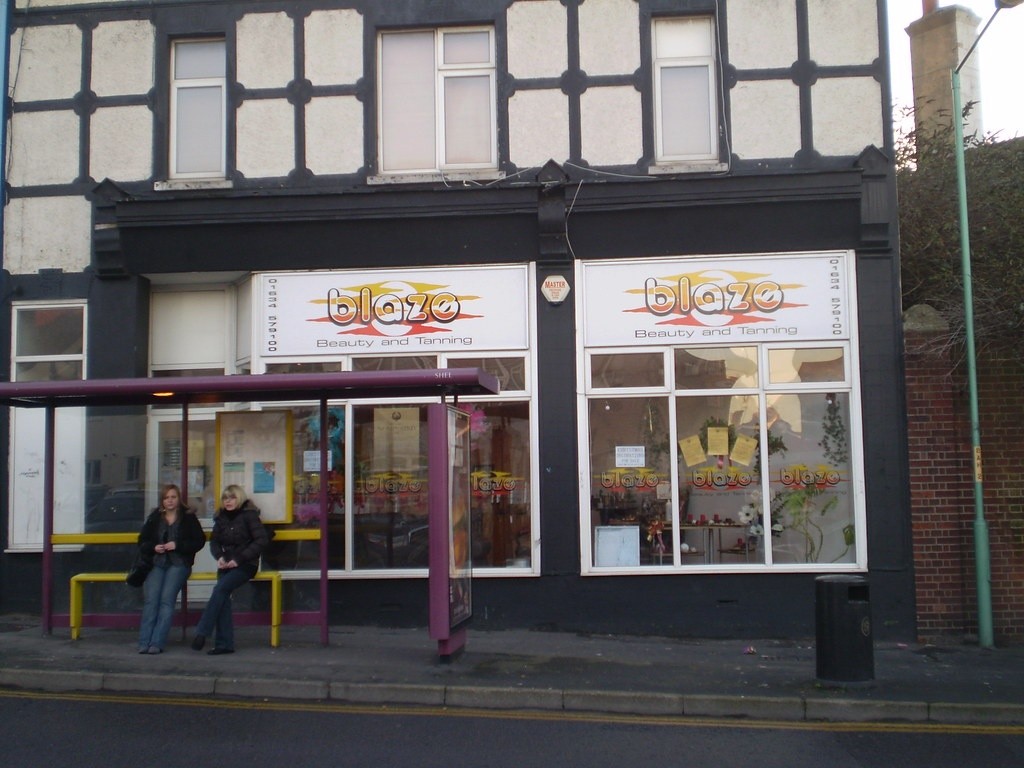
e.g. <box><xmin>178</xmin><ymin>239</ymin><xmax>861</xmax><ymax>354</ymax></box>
<box><xmin>70</xmin><ymin>572</ymin><xmax>282</xmax><ymax>647</ymax></box>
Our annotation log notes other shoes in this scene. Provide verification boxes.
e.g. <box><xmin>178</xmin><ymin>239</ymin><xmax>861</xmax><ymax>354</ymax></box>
<box><xmin>206</xmin><ymin>646</ymin><xmax>234</xmax><ymax>655</ymax></box>
<box><xmin>149</xmin><ymin>647</ymin><xmax>161</xmax><ymax>654</ymax></box>
<box><xmin>191</xmin><ymin>634</ymin><xmax>206</xmax><ymax>651</ymax></box>
<box><xmin>138</xmin><ymin>646</ymin><xmax>148</xmax><ymax>653</ymax></box>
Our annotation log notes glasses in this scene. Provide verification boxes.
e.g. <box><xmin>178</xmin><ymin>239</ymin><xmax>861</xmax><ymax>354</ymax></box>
<box><xmin>223</xmin><ymin>496</ymin><xmax>236</xmax><ymax>500</ymax></box>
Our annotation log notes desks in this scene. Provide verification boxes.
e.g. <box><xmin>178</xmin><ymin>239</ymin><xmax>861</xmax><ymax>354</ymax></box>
<box><xmin>664</xmin><ymin>523</ymin><xmax>745</xmax><ymax>564</ymax></box>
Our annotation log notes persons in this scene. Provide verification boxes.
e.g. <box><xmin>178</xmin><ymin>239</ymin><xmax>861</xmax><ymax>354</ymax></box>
<box><xmin>192</xmin><ymin>485</ymin><xmax>269</xmax><ymax>655</ymax></box>
<box><xmin>138</xmin><ymin>484</ymin><xmax>206</xmax><ymax>655</ymax></box>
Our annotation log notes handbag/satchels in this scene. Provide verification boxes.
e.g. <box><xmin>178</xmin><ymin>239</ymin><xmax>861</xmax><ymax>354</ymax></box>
<box><xmin>126</xmin><ymin>543</ymin><xmax>154</xmax><ymax>588</ymax></box>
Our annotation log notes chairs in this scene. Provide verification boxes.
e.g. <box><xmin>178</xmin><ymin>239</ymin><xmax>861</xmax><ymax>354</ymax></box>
<box><xmin>718</xmin><ymin>526</ymin><xmax>755</xmax><ymax>564</ymax></box>
<box><xmin>650</xmin><ymin>512</ymin><xmax>673</xmax><ymax>565</ymax></box>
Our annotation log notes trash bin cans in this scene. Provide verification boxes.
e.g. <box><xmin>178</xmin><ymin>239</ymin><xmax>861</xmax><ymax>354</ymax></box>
<box><xmin>815</xmin><ymin>575</ymin><xmax>875</xmax><ymax>683</ymax></box>
